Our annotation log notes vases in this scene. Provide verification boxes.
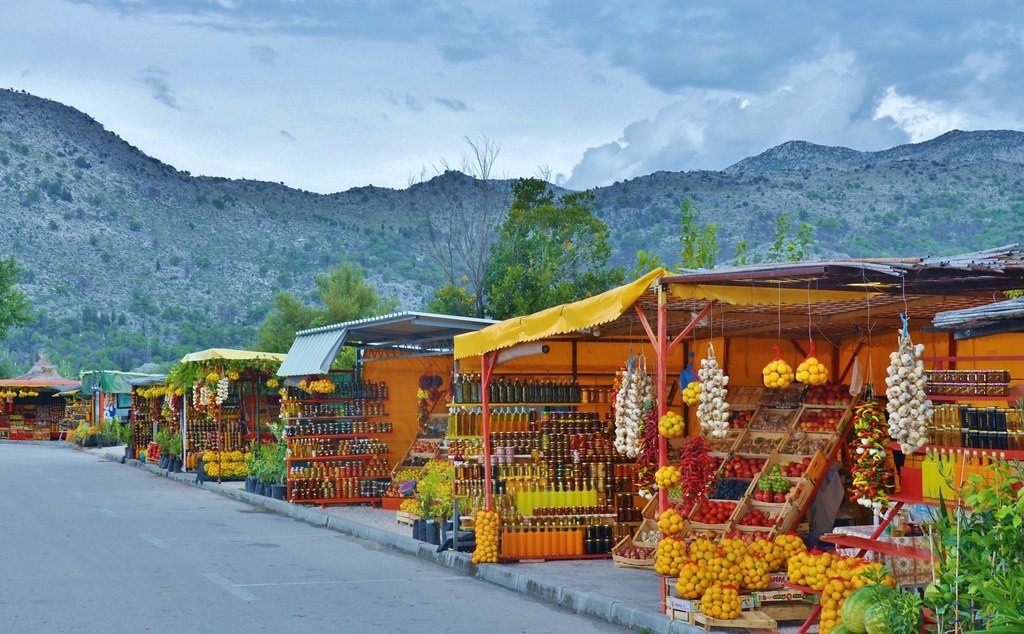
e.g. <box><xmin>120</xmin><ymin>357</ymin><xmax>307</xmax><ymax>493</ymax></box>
<box><xmin>417</xmin><ymin>519</ymin><xmax>426</xmax><ymax>541</ymax></box>
<box><xmin>413</xmin><ymin>519</ymin><xmax>419</xmax><ymax>540</ymax></box>
<box><xmin>426</xmin><ymin>522</ymin><xmax>441</xmax><ymax>545</ymax></box>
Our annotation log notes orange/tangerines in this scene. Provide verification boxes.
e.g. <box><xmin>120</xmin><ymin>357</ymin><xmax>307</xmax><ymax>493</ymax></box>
<box><xmin>202</xmin><ymin>450</ymin><xmax>252</xmax><ymax>477</ymax></box>
<box><xmin>762</xmin><ymin>357</ymin><xmax>828</xmax><ymax>388</ymax></box>
<box><xmin>136</xmin><ymin>369</ymin><xmax>239</xmax><ymax>398</ymax></box>
<box><xmin>472</xmin><ymin>510</ymin><xmax>501</xmax><ymax>563</ymax></box>
<box><xmin>267</xmin><ymin>378</ymin><xmax>336</xmax><ymax>396</ymax></box>
<box><xmin>653</xmin><ymin>508</ymin><xmax>898</xmax><ymax>634</ymax></box>
<box><xmin>655</xmin><ymin>382</ymin><xmax>702</xmax><ymax>489</ymax></box>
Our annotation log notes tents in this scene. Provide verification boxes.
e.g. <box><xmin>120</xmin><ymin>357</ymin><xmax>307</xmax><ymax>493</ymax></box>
<box><xmin>453</xmin><ymin>256</ymin><xmax>1024</xmax><ymax>615</ymax></box>
<box><xmin>0</xmin><ymin>310</ymin><xmax>502</xmax><ymax>509</ymax></box>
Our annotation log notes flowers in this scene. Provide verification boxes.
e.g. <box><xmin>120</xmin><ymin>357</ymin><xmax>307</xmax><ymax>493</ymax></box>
<box><xmin>414</xmin><ymin>460</ymin><xmax>455</xmax><ymax>520</ymax></box>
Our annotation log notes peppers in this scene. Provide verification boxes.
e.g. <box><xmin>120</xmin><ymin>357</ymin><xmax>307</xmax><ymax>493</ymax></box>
<box><xmin>634</xmin><ymin>398</ymin><xmax>659</xmax><ymax>496</ymax></box>
<box><xmin>848</xmin><ymin>395</ymin><xmax>896</xmax><ymax>510</ymax></box>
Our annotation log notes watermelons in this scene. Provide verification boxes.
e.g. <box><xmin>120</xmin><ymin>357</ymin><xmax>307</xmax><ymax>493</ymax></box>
<box><xmin>827</xmin><ymin>583</ymin><xmax>925</xmax><ymax>634</ymax></box>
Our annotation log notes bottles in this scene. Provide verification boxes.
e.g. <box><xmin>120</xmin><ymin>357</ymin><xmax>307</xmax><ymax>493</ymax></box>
<box><xmin>287</xmin><ymin>398</ymin><xmax>384</xmax><ymax>416</ymax></box>
<box><xmin>447</xmin><ymin>407</ymin><xmax>529</xmax><ymax>437</ymax></box>
<box><xmin>452</xmin><ymin>371</ymin><xmax>580</xmax><ymax>403</ymax></box>
<box><xmin>293</xmin><ymin>379</ymin><xmax>388</xmax><ymax>399</ymax></box>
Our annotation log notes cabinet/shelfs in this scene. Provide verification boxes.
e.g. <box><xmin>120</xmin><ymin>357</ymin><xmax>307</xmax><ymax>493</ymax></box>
<box><xmin>819</xmin><ymin>389</ymin><xmax>1024</xmax><ymax>563</ymax></box>
<box><xmin>279</xmin><ymin>397</ymin><xmax>396</xmax><ymax>509</ymax></box>
<box><xmin>444</xmin><ymin>402</ymin><xmax>642</xmax><ymax>562</ymax></box>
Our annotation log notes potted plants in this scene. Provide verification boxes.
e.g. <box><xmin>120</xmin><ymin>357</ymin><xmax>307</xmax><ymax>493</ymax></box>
<box><xmin>169</xmin><ymin>430</ymin><xmax>183</xmax><ymax>473</ymax></box>
<box><xmin>252</xmin><ymin>460</ymin><xmax>265</xmax><ymax>495</ymax></box>
<box><xmin>97</xmin><ymin>417</ymin><xmax>131</xmax><ymax>449</ymax></box>
<box><xmin>271</xmin><ymin>440</ymin><xmax>288</xmax><ymax>500</ymax></box>
<box><xmin>248</xmin><ymin>461</ymin><xmax>257</xmax><ymax>492</ymax></box>
<box><xmin>168</xmin><ymin>458</ymin><xmax>175</xmax><ymax>472</ymax></box>
<box><xmin>123</xmin><ymin>430</ymin><xmax>135</xmax><ymax>459</ymax></box>
<box><xmin>245</xmin><ymin>436</ymin><xmax>258</xmax><ymax>491</ymax></box>
<box><xmin>154</xmin><ymin>428</ymin><xmax>172</xmax><ymax>469</ymax></box>
<box><xmin>101</xmin><ymin>415</ymin><xmax>131</xmax><ymax>458</ymax></box>
<box><xmin>260</xmin><ymin>445</ymin><xmax>276</xmax><ymax>496</ymax></box>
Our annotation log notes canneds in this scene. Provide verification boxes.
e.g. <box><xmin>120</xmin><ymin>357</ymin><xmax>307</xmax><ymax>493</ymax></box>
<box><xmin>451</xmin><ymin>388</ymin><xmax>644</xmax><ymax>554</ymax></box>
<box><xmin>284</xmin><ymin>398</ymin><xmax>393</xmax><ymax>500</ymax></box>
<box><xmin>922</xmin><ymin>369</ymin><xmax>1024</xmax><ymax>450</ymax></box>
<box><xmin>890</xmin><ymin>508</ymin><xmax>922</xmax><ymax>536</ymax></box>
<box><xmin>134</xmin><ymin>413</ymin><xmax>245</xmax><ymax>452</ymax></box>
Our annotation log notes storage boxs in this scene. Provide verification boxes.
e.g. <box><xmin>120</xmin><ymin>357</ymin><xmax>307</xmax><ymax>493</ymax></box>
<box><xmin>631</xmin><ymin>518</ymin><xmax>684</xmax><ymax>545</ymax></box>
<box><xmin>611</xmin><ymin>535</ymin><xmax>657</xmax><ymax>570</ymax></box>
<box><xmin>689</xmin><ymin>499</ymin><xmax>741</xmax><ymax>524</ymax></box>
<box><xmin>390</xmin><ymin>391</ymin><xmax>450</xmax><ymax>483</ymax></box>
<box><xmin>704</xmin><ymin>471</ymin><xmax>757</xmax><ymax>501</ymax></box>
<box><xmin>749</xmin><ymin>471</ymin><xmax>817</xmax><ymax>514</ymax></box>
<box><xmin>718</xmin><ymin>451</ymin><xmax>772</xmax><ymax>478</ymax></box>
<box><xmin>751</xmin><ymin>589</ymin><xmax>806</xmax><ymax>602</ymax></box>
<box><xmin>758</xmin><ymin>382</ymin><xmax>809</xmax><ymax>409</ymax></box>
<box><xmin>408</xmin><ymin>512</ymin><xmax>422</xmax><ymax>526</ymax></box>
<box><xmin>726</xmin><ymin>406</ymin><xmax>757</xmax><ymax>431</ymax></box>
<box><xmin>764</xmin><ymin>449</ymin><xmax>827</xmax><ymax>480</ymax></box>
<box><xmin>768</xmin><ymin>572</ymin><xmax>789</xmax><ymax>589</ymax></box>
<box><xmin>724</xmin><ymin>521</ymin><xmax>782</xmax><ymax>541</ymax></box>
<box><xmin>802</xmin><ymin>382</ymin><xmax>863</xmax><ymax>409</ymax></box>
<box><xmin>733</xmin><ymin>496</ymin><xmax>799</xmax><ymax>534</ymax></box>
<box><xmin>641</xmin><ymin>492</ymin><xmax>698</xmax><ymax>521</ymax></box>
<box><xmin>734</xmin><ymin>428</ymin><xmax>788</xmax><ymax>455</ymax></box>
<box><xmin>396</xmin><ymin>510</ymin><xmax>409</xmax><ymax>524</ymax></box>
<box><xmin>779</xmin><ymin>432</ymin><xmax>840</xmax><ymax>460</ymax></box>
<box><xmin>699</xmin><ymin>428</ymin><xmax>744</xmax><ymax>452</ymax></box>
<box><xmin>792</xmin><ymin>406</ymin><xmax>854</xmax><ymax>435</ymax></box>
<box><xmin>741</xmin><ymin>594</ymin><xmax>754</xmax><ymax>608</ymax></box>
<box><xmin>665</xmin><ymin>596</ymin><xmax>701</xmax><ymax>613</ymax></box>
<box><xmin>676</xmin><ymin>520</ymin><xmax>728</xmax><ymax>541</ymax></box>
<box><xmin>747</xmin><ymin>405</ymin><xmax>802</xmax><ymax>433</ymax></box>
<box><xmin>0</xmin><ymin>425</ymin><xmax>51</xmax><ymax>440</ymax></box>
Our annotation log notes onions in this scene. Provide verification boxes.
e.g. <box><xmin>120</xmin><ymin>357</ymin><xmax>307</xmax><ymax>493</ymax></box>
<box><xmin>616</xmin><ymin>546</ymin><xmax>654</xmax><ymax>559</ymax></box>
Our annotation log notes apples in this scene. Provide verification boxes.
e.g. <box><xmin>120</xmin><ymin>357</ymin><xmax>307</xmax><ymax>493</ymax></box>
<box><xmin>668</xmin><ymin>382</ymin><xmax>855</xmax><ymax>542</ymax></box>
<box><xmin>386</xmin><ymin>440</ymin><xmax>442</xmax><ymax>498</ymax></box>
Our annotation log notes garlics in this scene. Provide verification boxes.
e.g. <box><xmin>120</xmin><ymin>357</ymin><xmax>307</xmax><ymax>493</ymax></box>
<box><xmin>885</xmin><ymin>344</ymin><xmax>934</xmax><ymax>455</ymax></box>
<box><xmin>638</xmin><ymin>530</ymin><xmax>661</xmax><ymax>543</ymax></box>
<box><xmin>697</xmin><ymin>358</ymin><xmax>729</xmax><ymax>440</ymax></box>
<box><xmin>614</xmin><ymin>369</ymin><xmax>653</xmax><ymax>458</ymax></box>
<box><xmin>192</xmin><ymin>376</ymin><xmax>229</xmax><ymax>406</ymax></box>
